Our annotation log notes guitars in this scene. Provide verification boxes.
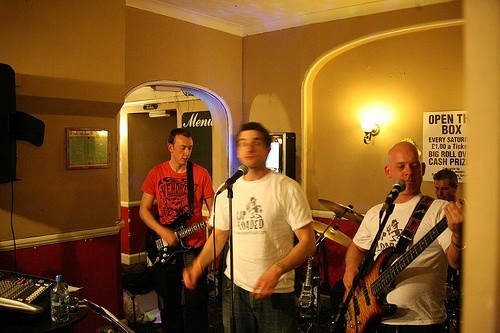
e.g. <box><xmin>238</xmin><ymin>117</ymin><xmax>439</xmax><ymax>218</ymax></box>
<box><xmin>297</xmin><ymin>254</ymin><xmax>324</xmax><ymax>320</ymax></box>
<box><xmin>342</xmin><ymin>197</ymin><xmax>465</xmax><ymax>333</ymax></box>
<box><xmin>144</xmin><ymin>211</ymin><xmax>209</xmax><ymax>268</ymax></box>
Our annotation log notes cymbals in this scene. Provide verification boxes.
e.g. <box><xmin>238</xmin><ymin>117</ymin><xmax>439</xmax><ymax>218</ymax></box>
<box><xmin>312</xmin><ymin>220</ymin><xmax>352</xmax><ymax>248</ymax></box>
<box><xmin>318</xmin><ymin>198</ymin><xmax>365</xmax><ymax>225</ymax></box>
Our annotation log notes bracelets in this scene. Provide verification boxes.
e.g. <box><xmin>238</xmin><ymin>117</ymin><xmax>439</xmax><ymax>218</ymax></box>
<box><xmin>451</xmin><ymin>239</ymin><xmax>465</xmax><ymax>250</ymax></box>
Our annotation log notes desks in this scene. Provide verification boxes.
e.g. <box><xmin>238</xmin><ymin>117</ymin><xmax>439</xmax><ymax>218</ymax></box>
<box><xmin>0</xmin><ymin>296</ymin><xmax>88</xmax><ymax>333</ymax></box>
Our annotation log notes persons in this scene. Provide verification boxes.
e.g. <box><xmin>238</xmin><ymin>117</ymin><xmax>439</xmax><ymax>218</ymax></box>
<box><xmin>139</xmin><ymin>128</ymin><xmax>215</xmax><ymax>333</ymax></box>
<box><xmin>343</xmin><ymin>138</ymin><xmax>466</xmax><ymax>333</ymax></box>
<box><xmin>433</xmin><ymin>169</ymin><xmax>459</xmax><ymax>201</ymax></box>
<box><xmin>183</xmin><ymin>122</ymin><xmax>315</xmax><ymax>333</ymax></box>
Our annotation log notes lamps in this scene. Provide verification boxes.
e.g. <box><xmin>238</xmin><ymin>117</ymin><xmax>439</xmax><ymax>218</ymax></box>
<box><xmin>358</xmin><ymin>102</ymin><xmax>379</xmax><ymax>144</ymax></box>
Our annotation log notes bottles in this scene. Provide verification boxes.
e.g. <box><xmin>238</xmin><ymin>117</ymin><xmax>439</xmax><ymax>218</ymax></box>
<box><xmin>50</xmin><ymin>275</ymin><xmax>69</xmax><ymax>323</ymax></box>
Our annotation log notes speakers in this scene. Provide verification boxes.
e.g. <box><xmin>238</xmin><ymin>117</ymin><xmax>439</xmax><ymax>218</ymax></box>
<box><xmin>0</xmin><ymin>62</ymin><xmax>17</xmax><ymax>183</ymax></box>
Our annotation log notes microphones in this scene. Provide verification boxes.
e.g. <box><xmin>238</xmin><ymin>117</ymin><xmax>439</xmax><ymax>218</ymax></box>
<box><xmin>216</xmin><ymin>165</ymin><xmax>249</xmax><ymax>195</ymax></box>
<box><xmin>381</xmin><ymin>179</ymin><xmax>406</xmax><ymax>211</ymax></box>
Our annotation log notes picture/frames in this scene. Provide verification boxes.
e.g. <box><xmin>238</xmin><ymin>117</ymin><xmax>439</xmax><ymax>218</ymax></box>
<box><xmin>66</xmin><ymin>126</ymin><xmax>112</xmax><ymax>170</ymax></box>
